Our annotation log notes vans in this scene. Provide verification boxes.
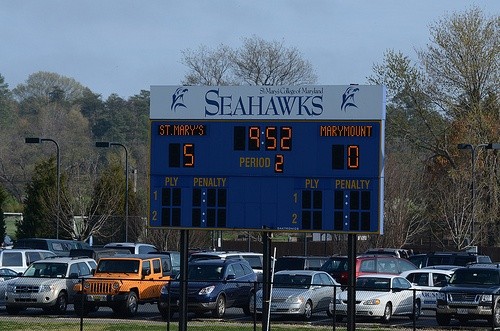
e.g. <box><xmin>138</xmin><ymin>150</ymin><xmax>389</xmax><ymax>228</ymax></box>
<box><xmin>0</xmin><ymin>249</ymin><xmax>60</xmax><ymax>277</ymax></box>
<box><xmin>11</xmin><ymin>239</ymin><xmax>95</xmax><ymax>258</ymax></box>
<box><xmin>102</xmin><ymin>242</ymin><xmax>160</xmax><ymax>255</ymax></box>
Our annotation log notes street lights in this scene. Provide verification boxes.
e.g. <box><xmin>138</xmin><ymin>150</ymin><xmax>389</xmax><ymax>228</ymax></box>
<box><xmin>457</xmin><ymin>142</ymin><xmax>500</xmax><ymax>253</ymax></box>
<box><xmin>95</xmin><ymin>141</ymin><xmax>128</xmax><ymax>243</ymax></box>
<box><xmin>24</xmin><ymin>137</ymin><xmax>60</xmax><ymax>239</ymax></box>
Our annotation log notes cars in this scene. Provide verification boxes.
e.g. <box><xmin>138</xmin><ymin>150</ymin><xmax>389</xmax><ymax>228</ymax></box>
<box><xmin>249</xmin><ymin>269</ymin><xmax>342</xmax><ymax>321</ymax></box>
<box><xmin>328</xmin><ymin>274</ymin><xmax>424</xmax><ymax>324</ymax></box>
<box><xmin>398</xmin><ymin>268</ymin><xmax>454</xmax><ymax>309</ymax></box>
<box><xmin>0</xmin><ymin>268</ymin><xmax>25</xmax><ymax>307</ymax></box>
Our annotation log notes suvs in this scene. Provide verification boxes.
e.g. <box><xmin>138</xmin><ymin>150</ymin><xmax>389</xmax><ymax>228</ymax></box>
<box><xmin>320</xmin><ymin>253</ymin><xmax>419</xmax><ymax>286</ymax></box>
<box><xmin>69</xmin><ymin>248</ymin><xmax>134</xmax><ymax>265</ymax></box>
<box><xmin>363</xmin><ymin>247</ymin><xmax>492</xmax><ymax>273</ymax></box>
<box><xmin>273</xmin><ymin>255</ymin><xmax>330</xmax><ymax>273</ymax></box>
<box><xmin>188</xmin><ymin>250</ymin><xmax>264</xmax><ymax>276</ymax></box>
<box><xmin>72</xmin><ymin>253</ymin><xmax>172</xmax><ymax>319</ymax></box>
<box><xmin>4</xmin><ymin>255</ymin><xmax>98</xmax><ymax>315</ymax></box>
<box><xmin>157</xmin><ymin>258</ymin><xmax>257</xmax><ymax>320</ymax></box>
<box><xmin>139</xmin><ymin>251</ymin><xmax>180</xmax><ymax>279</ymax></box>
<box><xmin>436</xmin><ymin>262</ymin><xmax>500</xmax><ymax>328</ymax></box>
